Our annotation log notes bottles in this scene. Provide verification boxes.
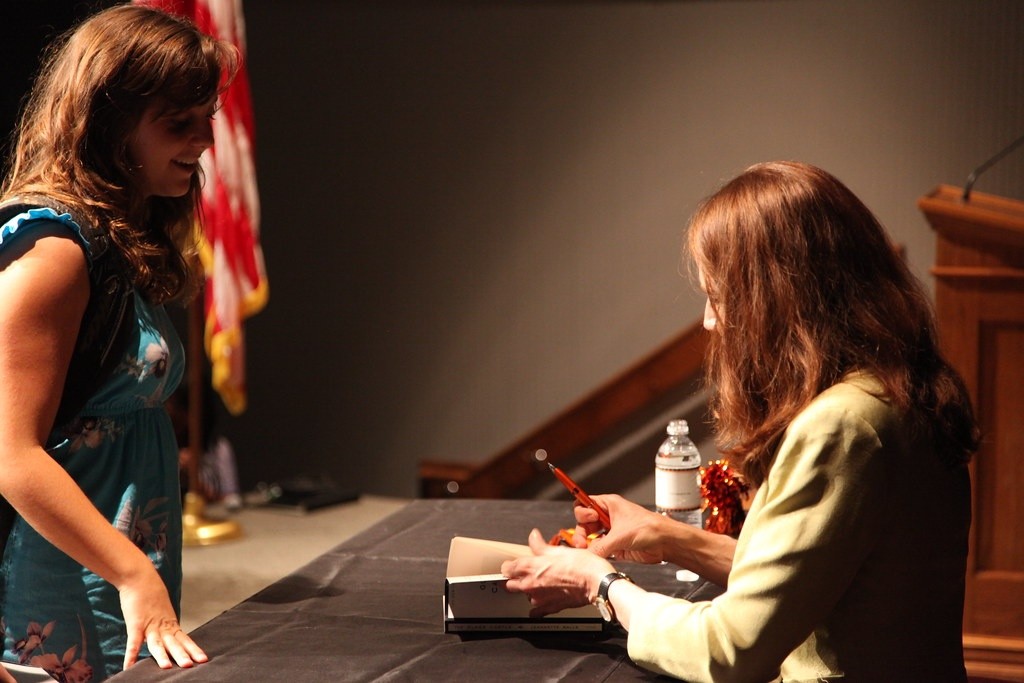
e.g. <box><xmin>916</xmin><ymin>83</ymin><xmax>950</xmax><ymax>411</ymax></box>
<box><xmin>654</xmin><ymin>419</ymin><xmax>703</xmax><ymax>530</ymax></box>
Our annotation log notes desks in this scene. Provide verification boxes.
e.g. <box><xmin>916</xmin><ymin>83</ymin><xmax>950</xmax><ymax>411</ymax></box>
<box><xmin>109</xmin><ymin>499</ymin><xmax>725</xmax><ymax>683</ymax></box>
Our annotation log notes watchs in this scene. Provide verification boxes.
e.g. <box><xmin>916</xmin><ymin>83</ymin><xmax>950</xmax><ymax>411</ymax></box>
<box><xmin>596</xmin><ymin>572</ymin><xmax>634</xmax><ymax>622</ymax></box>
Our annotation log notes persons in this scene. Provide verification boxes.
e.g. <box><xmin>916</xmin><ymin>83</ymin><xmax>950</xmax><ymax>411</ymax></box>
<box><xmin>502</xmin><ymin>162</ymin><xmax>979</xmax><ymax>683</ymax></box>
<box><xmin>0</xmin><ymin>5</ymin><xmax>239</xmax><ymax>683</ymax></box>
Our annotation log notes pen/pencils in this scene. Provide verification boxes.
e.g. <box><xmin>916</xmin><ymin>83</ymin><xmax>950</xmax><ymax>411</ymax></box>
<box><xmin>546</xmin><ymin>461</ymin><xmax>611</xmax><ymax>530</ymax></box>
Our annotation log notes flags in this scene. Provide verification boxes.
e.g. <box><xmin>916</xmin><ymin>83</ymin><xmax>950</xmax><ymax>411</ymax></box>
<box><xmin>134</xmin><ymin>0</ymin><xmax>270</xmax><ymax>417</ymax></box>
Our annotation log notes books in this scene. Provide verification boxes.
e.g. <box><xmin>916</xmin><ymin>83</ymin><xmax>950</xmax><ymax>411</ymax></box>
<box><xmin>443</xmin><ymin>534</ymin><xmax>609</xmax><ymax>634</ymax></box>
